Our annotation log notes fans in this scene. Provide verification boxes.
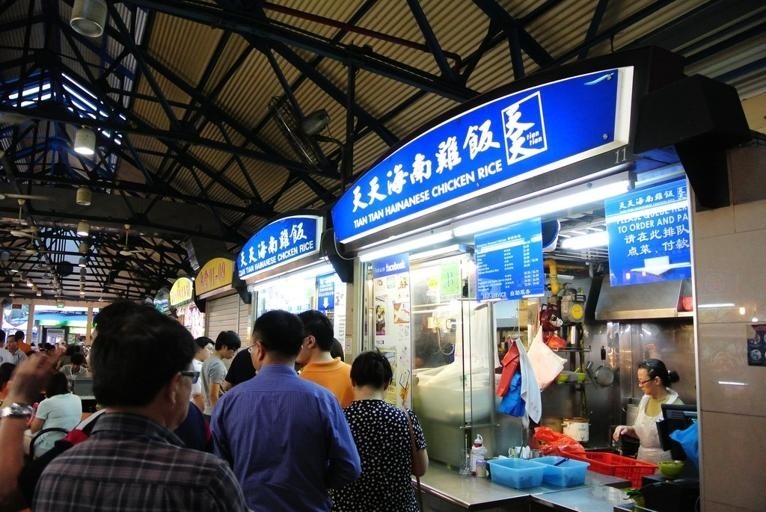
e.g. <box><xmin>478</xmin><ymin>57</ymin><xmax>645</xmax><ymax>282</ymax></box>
<box><xmin>268</xmin><ymin>96</ymin><xmax>344</xmax><ymax>176</ymax></box>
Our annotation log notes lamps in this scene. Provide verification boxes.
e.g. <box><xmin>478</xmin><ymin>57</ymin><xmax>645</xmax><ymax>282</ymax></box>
<box><xmin>8</xmin><ymin>219</ymin><xmax>90</xmax><ymax>297</ymax></box>
<box><xmin>119</xmin><ymin>224</ymin><xmax>132</xmax><ymax>256</ymax></box>
<box><xmin>75</xmin><ymin>185</ymin><xmax>92</xmax><ymax>206</ymax></box>
<box><xmin>73</xmin><ymin>125</ymin><xmax>96</xmax><ymax>161</ymax></box>
<box><xmin>69</xmin><ymin>0</ymin><xmax>108</xmax><ymax>38</ymax></box>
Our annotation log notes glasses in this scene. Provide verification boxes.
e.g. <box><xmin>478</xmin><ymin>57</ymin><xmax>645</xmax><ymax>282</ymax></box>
<box><xmin>182</xmin><ymin>371</ymin><xmax>199</xmax><ymax>384</ymax></box>
<box><xmin>247</xmin><ymin>343</ymin><xmax>257</xmax><ymax>353</ymax></box>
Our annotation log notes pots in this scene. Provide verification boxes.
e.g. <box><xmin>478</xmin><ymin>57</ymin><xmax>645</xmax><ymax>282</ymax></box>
<box><xmin>593</xmin><ymin>345</ymin><xmax>616</xmax><ymax>387</ymax></box>
<box><xmin>624</xmin><ymin>403</ymin><xmax>643</xmax><ymax>440</ymax></box>
<box><xmin>540</xmin><ymin>304</ymin><xmax>562</xmax><ymax>333</ymax></box>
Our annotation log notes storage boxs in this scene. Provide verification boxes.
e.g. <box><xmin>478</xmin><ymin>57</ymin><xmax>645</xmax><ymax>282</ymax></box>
<box><xmin>487</xmin><ymin>458</ymin><xmax>547</xmax><ymax>489</ymax></box>
<box><xmin>530</xmin><ymin>456</ymin><xmax>591</xmax><ymax>488</ymax></box>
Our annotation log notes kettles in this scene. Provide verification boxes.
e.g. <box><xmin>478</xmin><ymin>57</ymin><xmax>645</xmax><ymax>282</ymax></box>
<box><xmin>562</xmin><ymin>321</ymin><xmax>584</xmax><ymax>350</ymax></box>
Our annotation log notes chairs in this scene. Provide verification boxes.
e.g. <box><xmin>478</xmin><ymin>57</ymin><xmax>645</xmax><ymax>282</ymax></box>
<box><xmin>24</xmin><ymin>428</ymin><xmax>69</xmax><ymax>464</ymax></box>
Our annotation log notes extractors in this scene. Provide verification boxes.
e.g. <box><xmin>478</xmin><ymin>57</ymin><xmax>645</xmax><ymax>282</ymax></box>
<box><xmin>596</xmin><ymin>272</ymin><xmax>692</xmax><ymax>323</ymax></box>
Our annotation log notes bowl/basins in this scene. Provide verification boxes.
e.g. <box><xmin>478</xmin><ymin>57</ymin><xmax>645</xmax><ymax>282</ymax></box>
<box><xmin>555</xmin><ymin>374</ymin><xmax>569</xmax><ymax>385</ymax></box>
<box><xmin>656</xmin><ymin>459</ymin><xmax>685</xmax><ymax>477</ymax></box>
<box><xmin>575</xmin><ymin>373</ymin><xmax>586</xmax><ymax>384</ymax></box>
<box><xmin>562</xmin><ymin>370</ymin><xmax>580</xmax><ymax>383</ymax></box>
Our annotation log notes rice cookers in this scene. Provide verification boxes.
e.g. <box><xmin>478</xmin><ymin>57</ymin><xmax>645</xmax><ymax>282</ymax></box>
<box><xmin>560</xmin><ymin>416</ymin><xmax>593</xmax><ymax>445</ymax></box>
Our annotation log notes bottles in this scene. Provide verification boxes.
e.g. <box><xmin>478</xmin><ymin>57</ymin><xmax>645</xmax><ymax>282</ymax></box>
<box><xmin>475</xmin><ymin>459</ymin><xmax>487</xmax><ymax>479</ymax></box>
<box><xmin>470</xmin><ymin>433</ymin><xmax>486</xmax><ymax>476</ymax></box>
<box><xmin>507</xmin><ymin>441</ymin><xmax>545</xmax><ymax>460</ymax></box>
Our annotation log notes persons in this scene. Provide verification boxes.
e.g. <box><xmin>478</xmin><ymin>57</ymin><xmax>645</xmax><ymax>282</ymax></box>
<box><xmin>1</xmin><ymin>299</ymin><xmax>429</xmax><ymax>512</ymax></box>
<box><xmin>613</xmin><ymin>359</ymin><xmax>695</xmax><ymax>484</ymax></box>
